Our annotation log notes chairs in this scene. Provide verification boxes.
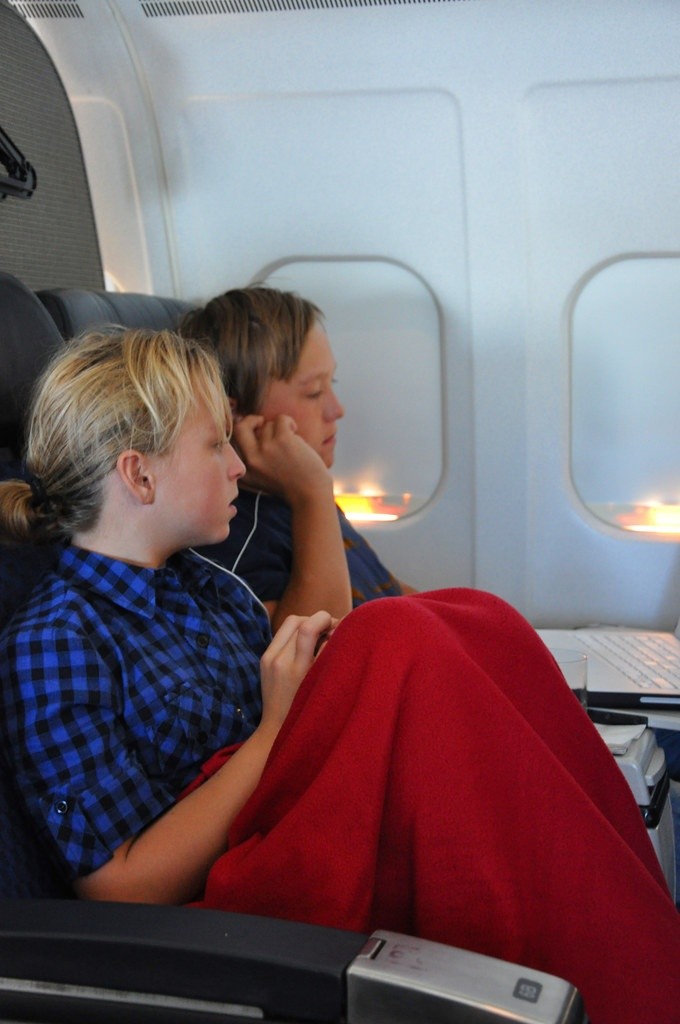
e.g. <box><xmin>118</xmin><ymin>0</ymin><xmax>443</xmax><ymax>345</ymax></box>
<box><xmin>0</xmin><ymin>268</ymin><xmax>675</xmax><ymax>1024</ymax></box>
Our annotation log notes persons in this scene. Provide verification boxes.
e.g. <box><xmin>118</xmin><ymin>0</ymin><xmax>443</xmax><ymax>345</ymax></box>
<box><xmin>173</xmin><ymin>288</ymin><xmax>421</xmax><ymax>653</ymax></box>
<box><xmin>0</xmin><ymin>322</ymin><xmax>348</xmax><ymax>904</ymax></box>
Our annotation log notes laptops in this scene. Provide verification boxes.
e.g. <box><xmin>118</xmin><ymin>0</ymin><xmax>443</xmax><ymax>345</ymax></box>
<box><xmin>533</xmin><ymin>617</ymin><xmax>680</xmax><ymax>709</ymax></box>
